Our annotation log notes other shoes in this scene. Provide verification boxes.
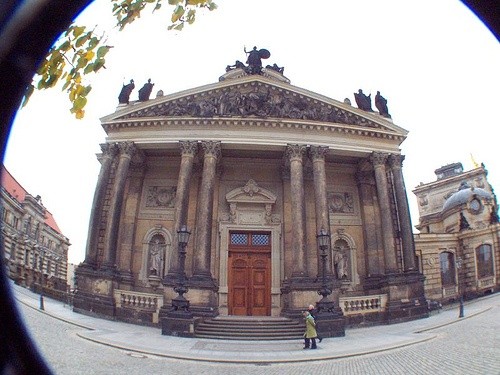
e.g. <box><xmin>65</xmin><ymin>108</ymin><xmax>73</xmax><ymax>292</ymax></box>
<box><xmin>303</xmin><ymin>346</ymin><xmax>310</xmax><ymax>350</ymax></box>
<box><xmin>311</xmin><ymin>346</ymin><xmax>317</xmax><ymax>350</ymax></box>
<box><xmin>319</xmin><ymin>337</ymin><xmax>322</xmax><ymax>343</ymax></box>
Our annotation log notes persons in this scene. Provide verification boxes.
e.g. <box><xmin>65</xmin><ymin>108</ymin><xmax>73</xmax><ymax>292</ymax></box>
<box><xmin>354</xmin><ymin>89</ymin><xmax>372</xmax><ymax>112</ymax></box>
<box><xmin>302</xmin><ymin>310</ymin><xmax>318</xmax><ymax>349</ymax></box>
<box><xmin>137</xmin><ymin>78</ymin><xmax>154</xmax><ymax>101</ymax></box>
<box><xmin>118</xmin><ymin>79</ymin><xmax>134</xmax><ymax>102</ymax></box>
<box><xmin>244</xmin><ymin>45</ymin><xmax>263</xmax><ymax>67</ymax></box>
<box><xmin>373</xmin><ymin>91</ymin><xmax>389</xmax><ymax>116</ymax></box>
<box><xmin>303</xmin><ymin>304</ymin><xmax>323</xmax><ymax>343</ymax></box>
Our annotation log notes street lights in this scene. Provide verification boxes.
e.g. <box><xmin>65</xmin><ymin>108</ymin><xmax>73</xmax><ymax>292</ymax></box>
<box><xmin>314</xmin><ymin>224</ymin><xmax>334</xmax><ymax>314</ymax></box>
<box><xmin>166</xmin><ymin>222</ymin><xmax>192</xmax><ymax>312</ymax></box>
